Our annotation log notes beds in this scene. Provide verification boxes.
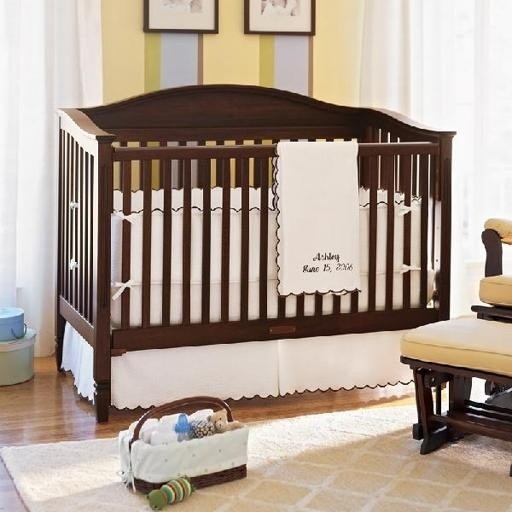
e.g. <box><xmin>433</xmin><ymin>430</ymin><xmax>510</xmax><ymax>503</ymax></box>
<box><xmin>49</xmin><ymin>80</ymin><xmax>457</xmax><ymax>426</ymax></box>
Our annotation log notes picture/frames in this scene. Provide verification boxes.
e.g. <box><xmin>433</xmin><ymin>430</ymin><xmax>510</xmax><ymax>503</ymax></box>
<box><xmin>142</xmin><ymin>0</ymin><xmax>221</xmax><ymax>36</ymax></box>
<box><xmin>242</xmin><ymin>2</ymin><xmax>316</xmax><ymax>38</ymax></box>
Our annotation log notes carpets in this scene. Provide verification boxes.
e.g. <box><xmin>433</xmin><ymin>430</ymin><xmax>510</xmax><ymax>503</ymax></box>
<box><xmin>1</xmin><ymin>402</ymin><xmax>512</xmax><ymax>511</ymax></box>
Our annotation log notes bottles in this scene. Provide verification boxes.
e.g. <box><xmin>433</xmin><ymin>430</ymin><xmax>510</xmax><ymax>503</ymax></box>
<box><xmin>174</xmin><ymin>414</ymin><xmax>191</xmax><ymax>443</ymax></box>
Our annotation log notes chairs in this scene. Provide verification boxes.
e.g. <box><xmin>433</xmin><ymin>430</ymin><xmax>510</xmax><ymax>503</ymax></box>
<box><xmin>400</xmin><ymin>214</ymin><xmax>512</xmax><ymax>475</ymax></box>
